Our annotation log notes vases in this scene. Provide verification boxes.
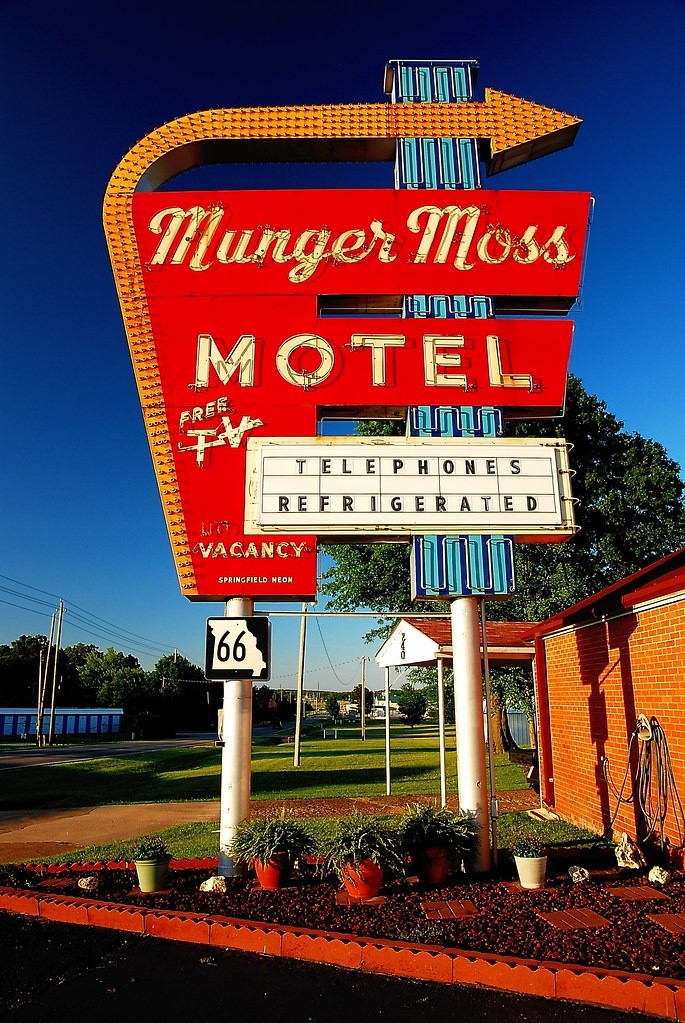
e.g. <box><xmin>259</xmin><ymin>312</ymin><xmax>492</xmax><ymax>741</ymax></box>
<box><xmin>514</xmin><ymin>856</ymin><xmax>547</xmax><ymax>890</ymax></box>
<box><xmin>134</xmin><ymin>859</ymin><xmax>169</xmax><ymax>893</ymax></box>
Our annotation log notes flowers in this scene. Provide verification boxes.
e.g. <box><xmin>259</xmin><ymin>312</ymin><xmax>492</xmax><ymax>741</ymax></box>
<box><xmin>510</xmin><ymin>829</ymin><xmax>549</xmax><ymax>857</ymax></box>
<box><xmin>129</xmin><ymin>836</ymin><xmax>170</xmax><ymax>860</ymax></box>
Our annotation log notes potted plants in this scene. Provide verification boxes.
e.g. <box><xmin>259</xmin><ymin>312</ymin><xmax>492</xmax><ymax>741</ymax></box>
<box><xmin>315</xmin><ymin>801</ymin><xmax>403</xmax><ymax>897</ymax></box>
<box><xmin>394</xmin><ymin>798</ymin><xmax>484</xmax><ymax>884</ymax></box>
<box><xmin>226</xmin><ymin>815</ymin><xmax>320</xmax><ymax>891</ymax></box>
